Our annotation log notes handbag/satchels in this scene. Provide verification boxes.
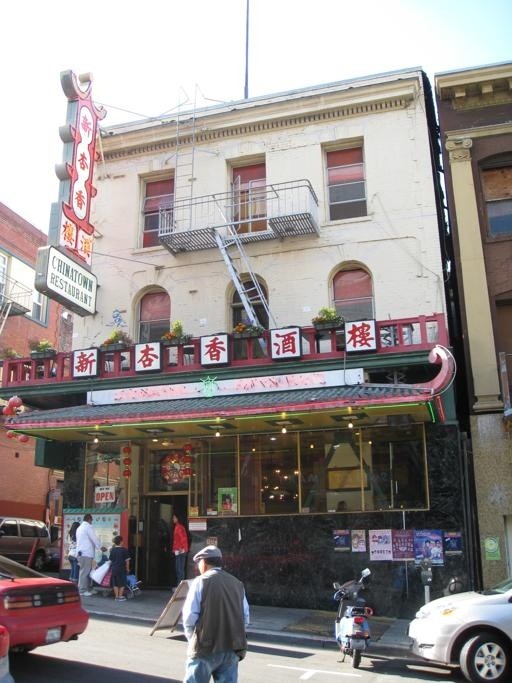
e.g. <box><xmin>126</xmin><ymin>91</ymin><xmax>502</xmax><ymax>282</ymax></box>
<box><xmin>100</xmin><ymin>568</ymin><xmax>113</xmax><ymax>588</ymax></box>
<box><xmin>88</xmin><ymin>559</ymin><xmax>113</xmax><ymax>585</ymax></box>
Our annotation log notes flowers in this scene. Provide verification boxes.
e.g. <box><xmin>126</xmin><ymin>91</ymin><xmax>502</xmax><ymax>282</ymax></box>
<box><xmin>161</xmin><ymin>320</ymin><xmax>183</xmax><ymax>340</ymax></box>
<box><xmin>98</xmin><ymin>329</ymin><xmax>133</xmax><ymax>345</ymax></box>
<box><xmin>232</xmin><ymin>319</ymin><xmax>265</xmax><ymax>337</ymax></box>
<box><xmin>0</xmin><ymin>347</ymin><xmax>22</xmax><ymax>361</ymax></box>
<box><xmin>29</xmin><ymin>340</ymin><xmax>58</xmax><ymax>353</ymax></box>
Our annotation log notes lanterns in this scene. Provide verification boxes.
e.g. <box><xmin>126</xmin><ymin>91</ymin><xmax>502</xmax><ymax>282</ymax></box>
<box><xmin>123</xmin><ymin>468</ymin><xmax>132</xmax><ymax>478</ymax></box>
<box><xmin>182</xmin><ymin>456</ymin><xmax>193</xmax><ymax>463</ymax></box>
<box><xmin>182</xmin><ymin>443</ymin><xmax>192</xmax><ymax>453</ymax></box>
<box><xmin>183</xmin><ymin>467</ymin><xmax>192</xmax><ymax>477</ymax></box>
<box><xmin>18</xmin><ymin>432</ymin><xmax>30</xmax><ymax>442</ymax></box>
<box><xmin>2</xmin><ymin>405</ymin><xmax>14</xmax><ymax>416</ymax></box>
<box><xmin>7</xmin><ymin>429</ymin><xmax>17</xmax><ymax>437</ymax></box>
<box><xmin>122</xmin><ymin>446</ymin><xmax>131</xmax><ymax>454</ymax></box>
<box><xmin>7</xmin><ymin>395</ymin><xmax>23</xmax><ymax>408</ymax></box>
<box><xmin>123</xmin><ymin>457</ymin><xmax>132</xmax><ymax>465</ymax></box>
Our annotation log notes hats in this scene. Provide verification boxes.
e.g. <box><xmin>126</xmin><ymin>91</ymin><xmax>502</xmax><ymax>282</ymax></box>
<box><xmin>192</xmin><ymin>545</ymin><xmax>222</xmax><ymax>563</ymax></box>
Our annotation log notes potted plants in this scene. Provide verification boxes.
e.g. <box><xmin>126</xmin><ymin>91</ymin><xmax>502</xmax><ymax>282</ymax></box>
<box><xmin>312</xmin><ymin>308</ymin><xmax>340</xmax><ymax>330</ymax></box>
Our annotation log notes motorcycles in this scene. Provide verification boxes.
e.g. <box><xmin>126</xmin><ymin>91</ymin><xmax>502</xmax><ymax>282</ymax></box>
<box><xmin>329</xmin><ymin>567</ymin><xmax>380</xmax><ymax>670</ymax></box>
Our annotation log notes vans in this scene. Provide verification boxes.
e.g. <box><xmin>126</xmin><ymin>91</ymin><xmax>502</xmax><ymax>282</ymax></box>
<box><xmin>1</xmin><ymin>516</ymin><xmax>50</xmax><ymax>575</ymax></box>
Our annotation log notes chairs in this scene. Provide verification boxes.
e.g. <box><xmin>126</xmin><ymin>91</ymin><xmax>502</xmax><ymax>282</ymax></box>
<box><xmin>100</xmin><ymin>344</ymin><xmax>125</xmax><ymax>374</ymax></box>
<box><xmin>183</xmin><ymin>333</ymin><xmax>194</xmax><ymax>365</ymax></box>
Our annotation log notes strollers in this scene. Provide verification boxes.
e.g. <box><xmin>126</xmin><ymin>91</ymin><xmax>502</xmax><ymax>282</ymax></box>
<box><xmin>92</xmin><ymin>547</ymin><xmax>143</xmax><ymax>599</ymax></box>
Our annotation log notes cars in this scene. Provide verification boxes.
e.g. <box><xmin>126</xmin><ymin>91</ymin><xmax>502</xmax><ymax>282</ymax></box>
<box><xmin>210</xmin><ymin>536</ymin><xmax>325</xmax><ymax>589</ymax></box>
<box><xmin>48</xmin><ymin>536</ymin><xmax>62</xmax><ymax>566</ymax></box>
<box><xmin>403</xmin><ymin>577</ymin><xmax>512</xmax><ymax>683</ymax></box>
<box><xmin>0</xmin><ymin>623</ymin><xmax>20</xmax><ymax>683</ymax></box>
<box><xmin>0</xmin><ymin>548</ymin><xmax>89</xmax><ymax>656</ymax></box>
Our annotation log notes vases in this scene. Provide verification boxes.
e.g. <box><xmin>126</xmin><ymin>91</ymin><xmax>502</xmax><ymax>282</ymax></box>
<box><xmin>30</xmin><ymin>352</ymin><xmax>57</xmax><ymax>358</ymax></box>
<box><xmin>100</xmin><ymin>343</ymin><xmax>127</xmax><ymax>352</ymax></box>
<box><xmin>0</xmin><ymin>361</ymin><xmax>3</xmax><ymax>367</ymax></box>
<box><xmin>162</xmin><ymin>337</ymin><xmax>187</xmax><ymax>346</ymax></box>
<box><xmin>233</xmin><ymin>332</ymin><xmax>251</xmax><ymax>338</ymax></box>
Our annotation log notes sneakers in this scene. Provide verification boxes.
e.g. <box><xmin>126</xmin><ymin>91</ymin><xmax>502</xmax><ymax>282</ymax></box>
<box><xmin>89</xmin><ymin>589</ymin><xmax>98</xmax><ymax>595</ymax></box>
<box><xmin>114</xmin><ymin>597</ymin><xmax>118</xmax><ymax>602</ymax></box>
<box><xmin>79</xmin><ymin>589</ymin><xmax>93</xmax><ymax>597</ymax></box>
<box><xmin>118</xmin><ymin>596</ymin><xmax>127</xmax><ymax>602</ymax></box>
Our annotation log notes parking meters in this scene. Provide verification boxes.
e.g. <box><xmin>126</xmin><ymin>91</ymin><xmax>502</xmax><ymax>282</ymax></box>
<box><xmin>417</xmin><ymin>555</ymin><xmax>434</xmax><ymax>612</ymax></box>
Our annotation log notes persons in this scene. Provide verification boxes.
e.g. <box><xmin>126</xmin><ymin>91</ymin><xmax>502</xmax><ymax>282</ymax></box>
<box><xmin>430</xmin><ymin>540</ymin><xmax>442</xmax><ymax>559</ymax></box>
<box><xmin>76</xmin><ymin>513</ymin><xmax>107</xmax><ymax>596</ymax></box>
<box><xmin>181</xmin><ymin>545</ymin><xmax>250</xmax><ymax>682</ymax></box>
<box><xmin>171</xmin><ymin>510</ymin><xmax>189</xmax><ymax>591</ymax></box>
<box><xmin>66</xmin><ymin>521</ymin><xmax>80</xmax><ymax>582</ymax></box>
<box><xmin>109</xmin><ymin>535</ymin><xmax>131</xmax><ymax>602</ymax></box>
<box><xmin>351</xmin><ymin>533</ymin><xmax>361</xmax><ymax>552</ymax></box>
<box><xmin>422</xmin><ymin>539</ymin><xmax>431</xmax><ymax>558</ymax></box>
